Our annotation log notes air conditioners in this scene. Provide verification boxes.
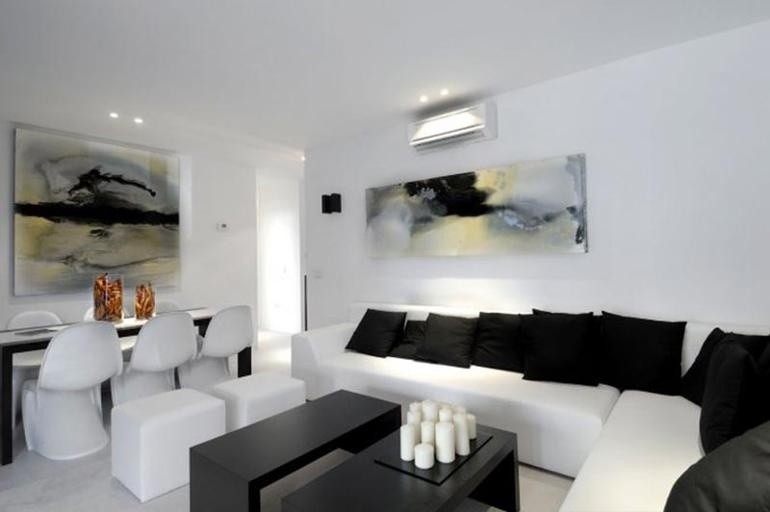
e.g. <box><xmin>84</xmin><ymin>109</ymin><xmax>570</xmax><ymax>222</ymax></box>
<box><xmin>408</xmin><ymin>100</ymin><xmax>497</xmax><ymax>154</ymax></box>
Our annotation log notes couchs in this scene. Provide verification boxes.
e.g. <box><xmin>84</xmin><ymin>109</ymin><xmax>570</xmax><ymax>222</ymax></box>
<box><xmin>288</xmin><ymin>298</ymin><xmax>770</xmax><ymax>511</ymax></box>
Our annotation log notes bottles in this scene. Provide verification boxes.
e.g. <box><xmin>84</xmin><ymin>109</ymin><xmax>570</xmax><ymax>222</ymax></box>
<box><xmin>400</xmin><ymin>398</ymin><xmax>477</xmax><ymax>469</ymax></box>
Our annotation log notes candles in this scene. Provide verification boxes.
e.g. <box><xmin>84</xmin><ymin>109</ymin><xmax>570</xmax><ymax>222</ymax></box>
<box><xmin>398</xmin><ymin>396</ymin><xmax>479</xmax><ymax>469</ymax></box>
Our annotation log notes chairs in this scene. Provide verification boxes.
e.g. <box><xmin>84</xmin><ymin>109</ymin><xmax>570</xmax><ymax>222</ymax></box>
<box><xmin>21</xmin><ymin>320</ymin><xmax>124</xmax><ymax>460</ymax></box>
<box><xmin>151</xmin><ymin>299</ymin><xmax>185</xmax><ymax>316</ymax></box>
<box><xmin>12</xmin><ymin>310</ymin><xmax>64</xmax><ymax>429</ymax></box>
<box><xmin>184</xmin><ymin>308</ymin><xmax>258</xmax><ymax>389</ymax></box>
<box><xmin>86</xmin><ymin>302</ymin><xmax>130</xmax><ymax>324</ymax></box>
<box><xmin>110</xmin><ymin>311</ymin><xmax>199</xmax><ymax>399</ymax></box>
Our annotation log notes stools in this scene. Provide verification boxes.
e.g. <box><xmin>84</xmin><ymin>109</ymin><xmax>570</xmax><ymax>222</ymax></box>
<box><xmin>208</xmin><ymin>371</ymin><xmax>307</xmax><ymax>434</ymax></box>
<box><xmin>109</xmin><ymin>389</ymin><xmax>227</xmax><ymax>505</ymax></box>
